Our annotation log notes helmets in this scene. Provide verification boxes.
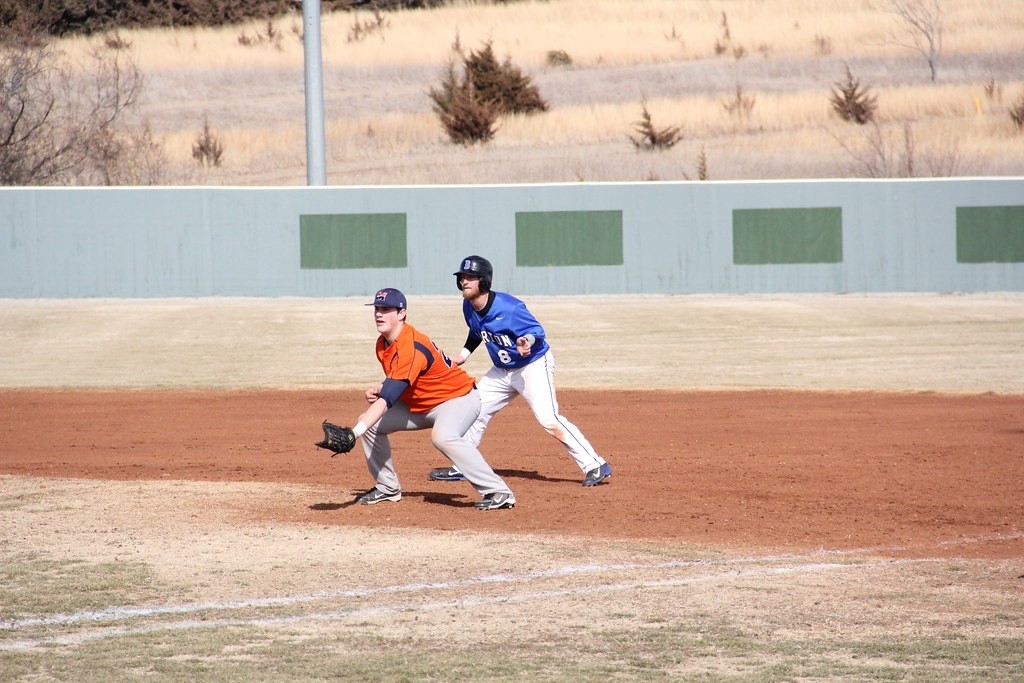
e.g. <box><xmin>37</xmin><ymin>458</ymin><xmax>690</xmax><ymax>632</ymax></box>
<box><xmin>453</xmin><ymin>255</ymin><xmax>493</xmax><ymax>284</ymax></box>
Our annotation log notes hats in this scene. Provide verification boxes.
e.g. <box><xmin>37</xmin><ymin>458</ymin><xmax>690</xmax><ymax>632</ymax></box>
<box><xmin>374</xmin><ymin>288</ymin><xmax>407</xmax><ymax>310</ymax></box>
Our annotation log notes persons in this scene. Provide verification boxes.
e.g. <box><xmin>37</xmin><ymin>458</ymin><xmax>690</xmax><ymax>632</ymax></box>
<box><xmin>314</xmin><ymin>287</ymin><xmax>516</xmax><ymax>511</ymax></box>
<box><xmin>428</xmin><ymin>255</ymin><xmax>612</xmax><ymax>488</ymax></box>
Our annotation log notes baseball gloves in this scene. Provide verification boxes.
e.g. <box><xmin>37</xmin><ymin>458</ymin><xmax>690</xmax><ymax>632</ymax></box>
<box><xmin>314</xmin><ymin>422</ymin><xmax>356</xmax><ymax>453</ymax></box>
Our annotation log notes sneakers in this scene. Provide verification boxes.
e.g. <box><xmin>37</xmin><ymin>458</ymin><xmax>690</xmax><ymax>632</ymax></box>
<box><xmin>355</xmin><ymin>486</ymin><xmax>402</xmax><ymax>505</ymax></box>
<box><xmin>475</xmin><ymin>493</ymin><xmax>517</xmax><ymax>511</ymax></box>
<box><xmin>582</xmin><ymin>462</ymin><xmax>612</xmax><ymax>487</ymax></box>
<box><xmin>429</xmin><ymin>466</ymin><xmax>468</xmax><ymax>481</ymax></box>
